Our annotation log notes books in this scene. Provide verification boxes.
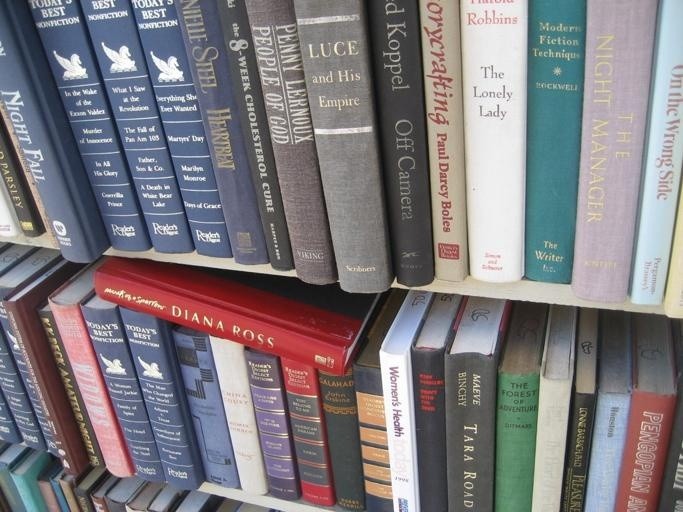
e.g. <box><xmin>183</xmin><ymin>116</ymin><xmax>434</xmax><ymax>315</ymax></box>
<box><xmin>2</xmin><ymin>246</ymin><xmax>682</xmax><ymax>510</ymax></box>
<box><xmin>0</xmin><ymin>1</ymin><xmax>679</xmax><ymax>318</ymax></box>
<box><xmin>93</xmin><ymin>259</ymin><xmax>384</xmax><ymax>374</ymax></box>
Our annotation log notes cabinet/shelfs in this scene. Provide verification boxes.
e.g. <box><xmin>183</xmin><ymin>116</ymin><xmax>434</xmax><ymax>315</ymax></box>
<box><xmin>0</xmin><ymin>228</ymin><xmax>667</xmax><ymax>512</ymax></box>
